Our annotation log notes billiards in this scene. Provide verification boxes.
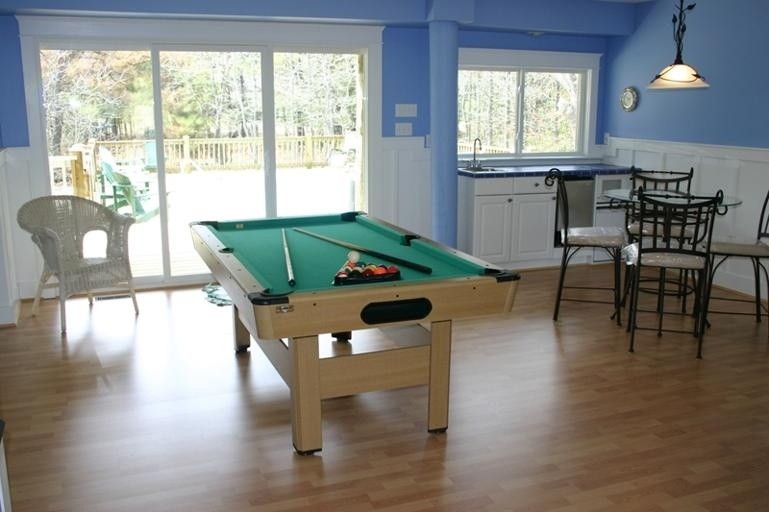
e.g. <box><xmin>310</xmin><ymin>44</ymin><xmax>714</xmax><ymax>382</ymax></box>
<box><xmin>337</xmin><ymin>263</ymin><xmax>399</xmax><ymax>277</ymax></box>
<box><xmin>348</xmin><ymin>251</ymin><xmax>359</xmax><ymax>263</ymax></box>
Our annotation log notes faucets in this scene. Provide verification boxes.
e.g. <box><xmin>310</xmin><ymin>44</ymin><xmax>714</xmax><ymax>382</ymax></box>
<box><xmin>474</xmin><ymin>138</ymin><xmax>481</xmax><ymax>163</ymax></box>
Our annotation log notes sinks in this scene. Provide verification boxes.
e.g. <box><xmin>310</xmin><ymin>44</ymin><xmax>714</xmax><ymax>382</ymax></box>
<box><xmin>463</xmin><ymin>169</ymin><xmax>504</xmax><ymax>172</ymax></box>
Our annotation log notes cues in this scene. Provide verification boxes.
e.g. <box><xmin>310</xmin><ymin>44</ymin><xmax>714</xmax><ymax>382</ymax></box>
<box><xmin>281</xmin><ymin>228</ymin><xmax>295</xmax><ymax>286</ymax></box>
<box><xmin>293</xmin><ymin>228</ymin><xmax>432</xmax><ymax>275</ymax></box>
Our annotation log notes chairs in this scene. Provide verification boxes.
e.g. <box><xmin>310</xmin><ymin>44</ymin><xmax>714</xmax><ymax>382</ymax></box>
<box><xmin>620</xmin><ymin>165</ymin><xmax>697</xmax><ymax>314</ymax></box>
<box><xmin>551</xmin><ymin>168</ymin><xmax>630</xmax><ymax>328</ymax></box>
<box><xmin>626</xmin><ymin>186</ymin><xmax>724</xmax><ymax>357</ymax></box>
<box><xmin>699</xmin><ymin>192</ymin><xmax>767</xmax><ymax>328</ymax></box>
<box><xmin>16</xmin><ymin>195</ymin><xmax>143</xmax><ymax>343</ymax></box>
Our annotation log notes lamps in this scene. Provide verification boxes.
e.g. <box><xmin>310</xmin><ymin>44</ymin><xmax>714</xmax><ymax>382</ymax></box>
<box><xmin>643</xmin><ymin>3</ymin><xmax>713</xmax><ymax>89</ymax></box>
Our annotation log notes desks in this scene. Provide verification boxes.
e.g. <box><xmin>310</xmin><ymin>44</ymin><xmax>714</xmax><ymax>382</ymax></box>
<box><xmin>604</xmin><ymin>188</ymin><xmax>741</xmax><ymax>336</ymax></box>
<box><xmin>189</xmin><ymin>210</ymin><xmax>521</xmax><ymax>455</ymax></box>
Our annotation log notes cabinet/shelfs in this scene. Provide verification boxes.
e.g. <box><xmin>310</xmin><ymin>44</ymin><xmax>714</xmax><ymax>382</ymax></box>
<box><xmin>594</xmin><ymin>173</ymin><xmax>632</xmax><ymax>267</ymax></box>
<box><xmin>513</xmin><ymin>176</ymin><xmax>557</xmax><ymax>268</ymax></box>
<box><xmin>455</xmin><ymin>173</ymin><xmax>513</xmax><ymax>274</ymax></box>
